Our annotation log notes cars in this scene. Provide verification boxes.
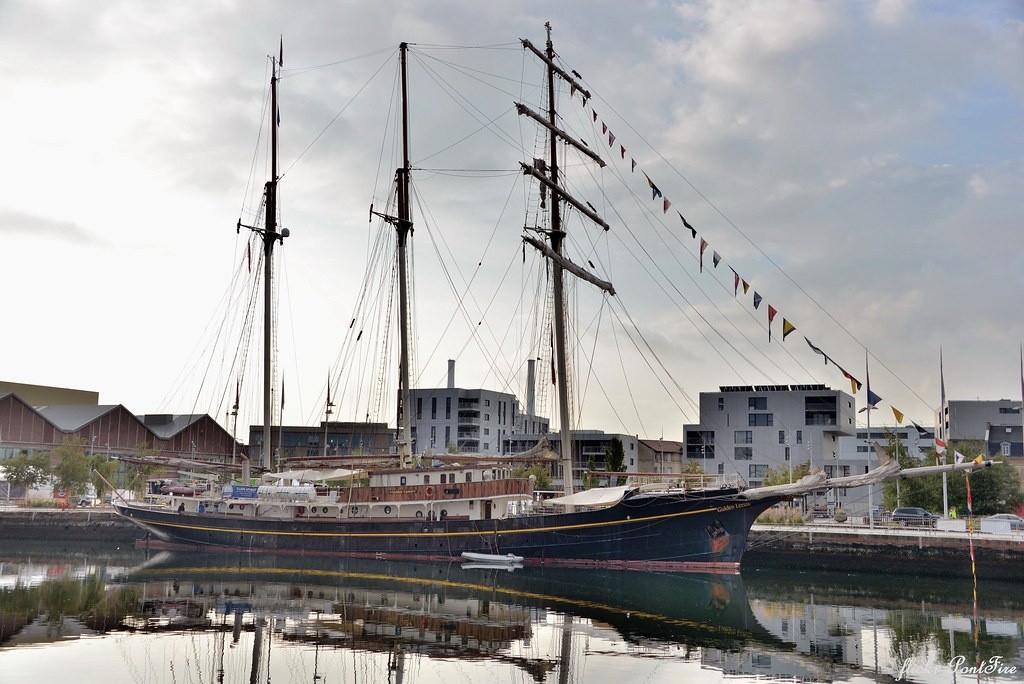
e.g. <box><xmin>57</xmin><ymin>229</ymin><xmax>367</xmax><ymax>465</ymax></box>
<box><xmin>75</xmin><ymin>495</ymin><xmax>97</xmax><ymax>506</ymax></box>
<box><xmin>990</xmin><ymin>513</ymin><xmax>1024</xmax><ymax>529</ymax></box>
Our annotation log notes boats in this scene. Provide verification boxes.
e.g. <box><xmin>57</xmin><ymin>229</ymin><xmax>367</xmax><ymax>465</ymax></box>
<box><xmin>461</xmin><ymin>562</ymin><xmax>524</xmax><ymax>573</ymax></box>
<box><xmin>460</xmin><ymin>551</ymin><xmax>525</xmax><ymax>563</ymax></box>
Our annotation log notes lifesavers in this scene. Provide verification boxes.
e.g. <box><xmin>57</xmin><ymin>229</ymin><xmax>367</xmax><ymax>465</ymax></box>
<box><xmin>425</xmin><ymin>486</ymin><xmax>434</xmax><ymax>496</ymax></box>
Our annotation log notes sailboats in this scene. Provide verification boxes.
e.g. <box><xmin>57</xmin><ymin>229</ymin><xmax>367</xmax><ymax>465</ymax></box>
<box><xmin>96</xmin><ymin>549</ymin><xmax>908</xmax><ymax>684</ymax></box>
<box><xmin>109</xmin><ymin>20</ymin><xmax>995</xmax><ymax>593</ymax></box>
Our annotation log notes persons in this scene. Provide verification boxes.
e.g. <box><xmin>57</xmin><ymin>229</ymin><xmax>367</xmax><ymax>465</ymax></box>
<box><xmin>177</xmin><ymin>502</ymin><xmax>185</xmax><ymax>513</ymax></box>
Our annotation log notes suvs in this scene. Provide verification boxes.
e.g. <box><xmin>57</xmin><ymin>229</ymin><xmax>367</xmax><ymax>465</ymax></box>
<box><xmin>891</xmin><ymin>507</ymin><xmax>942</xmax><ymax>528</ymax></box>
